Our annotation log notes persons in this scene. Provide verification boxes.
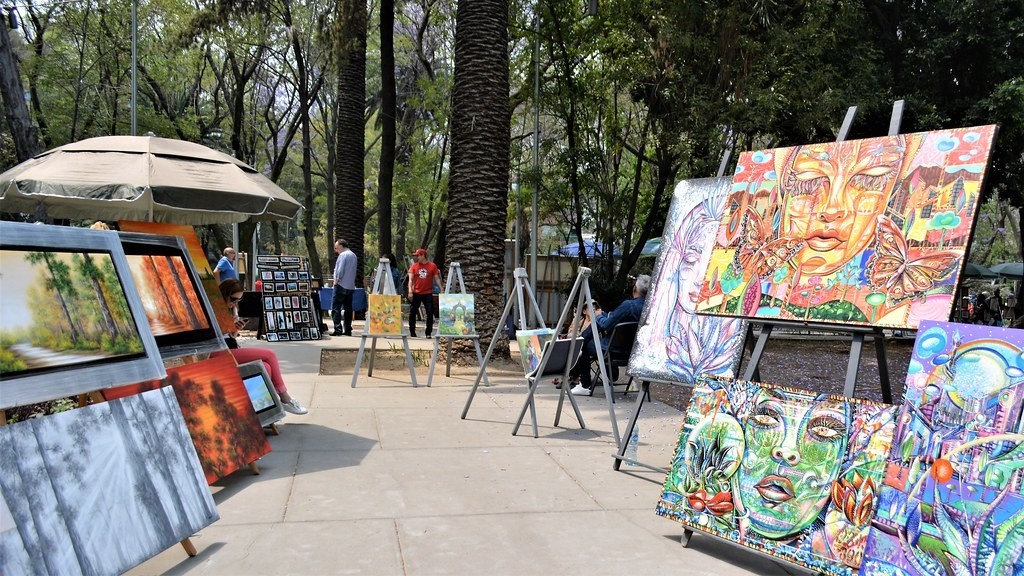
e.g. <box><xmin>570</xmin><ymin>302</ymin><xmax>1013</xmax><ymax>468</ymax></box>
<box><xmin>214</xmin><ymin>247</ymin><xmax>241</xmax><ymax>337</ymax></box>
<box><xmin>408</xmin><ymin>248</ymin><xmax>445</xmax><ymax>339</ymax></box>
<box><xmin>329</xmin><ymin>239</ymin><xmax>357</xmax><ymax>336</ymax></box>
<box><xmin>219</xmin><ymin>278</ymin><xmax>308</xmax><ymax>428</ymax></box>
<box><xmin>964</xmin><ymin>288</ymin><xmax>1024</xmax><ymax>329</ymax></box>
<box><xmin>564</xmin><ymin>274</ymin><xmax>651</xmax><ymax>395</ymax></box>
<box><xmin>554</xmin><ymin>299</ymin><xmax>601</xmax><ymax>389</ymax></box>
<box><xmin>372</xmin><ymin>253</ymin><xmax>402</xmax><ymax>296</ymax></box>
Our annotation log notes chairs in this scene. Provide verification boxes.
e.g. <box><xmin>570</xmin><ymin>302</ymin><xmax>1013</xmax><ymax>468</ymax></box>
<box><xmin>589</xmin><ymin>321</ymin><xmax>650</xmax><ymax>403</ymax></box>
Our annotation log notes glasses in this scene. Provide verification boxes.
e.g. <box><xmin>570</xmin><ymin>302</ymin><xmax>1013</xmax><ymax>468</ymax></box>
<box><xmin>230</xmin><ymin>296</ymin><xmax>243</xmax><ymax>302</ymax></box>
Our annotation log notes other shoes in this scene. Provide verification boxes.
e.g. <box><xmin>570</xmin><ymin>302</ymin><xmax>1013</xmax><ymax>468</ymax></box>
<box><xmin>556</xmin><ymin>380</ymin><xmax>579</xmax><ymax>389</ymax></box>
<box><xmin>329</xmin><ymin>332</ymin><xmax>342</xmax><ymax>336</ymax></box>
<box><xmin>426</xmin><ymin>335</ymin><xmax>431</xmax><ymax>339</ymax></box>
<box><xmin>342</xmin><ymin>332</ymin><xmax>353</xmax><ymax>336</ymax></box>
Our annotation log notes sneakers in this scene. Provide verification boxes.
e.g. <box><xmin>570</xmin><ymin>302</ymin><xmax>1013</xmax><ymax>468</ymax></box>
<box><xmin>566</xmin><ymin>383</ymin><xmax>592</xmax><ymax>395</ymax></box>
<box><xmin>280</xmin><ymin>399</ymin><xmax>308</xmax><ymax>415</ymax></box>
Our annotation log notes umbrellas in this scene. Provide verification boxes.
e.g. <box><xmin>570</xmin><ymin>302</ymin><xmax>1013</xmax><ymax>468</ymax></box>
<box><xmin>0</xmin><ymin>131</ymin><xmax>306</xmax><ymax>226</ymax></box>
<box><xmin>552</xmin><ymin>241</ymin><xmax>622</xmax><ymax>256</ymax></box>
<box><xmin>959</xmin><ymin>263</ymin><xmax>998</xmax><ymax>279</ymax></box>
<box><xmin>989</xmin><ymin>261</ymin><xmax>1024</xmax><ymax>296</ymax></box>
<box><xmin>627</xmin><ymin>237</ymin><xmax>663</xmax><ymax>255</ymax></box>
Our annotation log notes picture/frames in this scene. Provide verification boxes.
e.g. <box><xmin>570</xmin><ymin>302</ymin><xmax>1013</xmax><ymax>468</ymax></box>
<box><xmin>0</xmin><ymin>245</ymin><xmax>150</xmax><ymax>380</ymax></box>
<box><xmin>122</xmin><ymin>242</ymin><xmax>216</xmax><ymax>346</ymax></box>
<box><xmin>263</xmin><ymin>271</ymin><xmax>319</xmax><ymax>342</ymax></box>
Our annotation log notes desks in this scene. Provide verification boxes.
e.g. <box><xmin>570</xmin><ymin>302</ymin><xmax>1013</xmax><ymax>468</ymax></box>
<box><xmin>236</xmin><ymin>289</ymin><xmax>324</xmax><ymax>342</ymax></box>
<box><xmin>317</xmin><ymin>288</ymin><xmax>367</xmax><ymax>321</ymax></box>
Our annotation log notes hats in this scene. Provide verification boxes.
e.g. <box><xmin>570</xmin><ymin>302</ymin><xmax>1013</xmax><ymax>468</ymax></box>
<box><xmin>411</xmin><ymin>248</ymin><xmax>427</xmax><ymax>257</ymax></box>
<box><xmin>582</xmin><ymin>299</ymin><xmax>600</xmax><ymax>309</ymax></box>
